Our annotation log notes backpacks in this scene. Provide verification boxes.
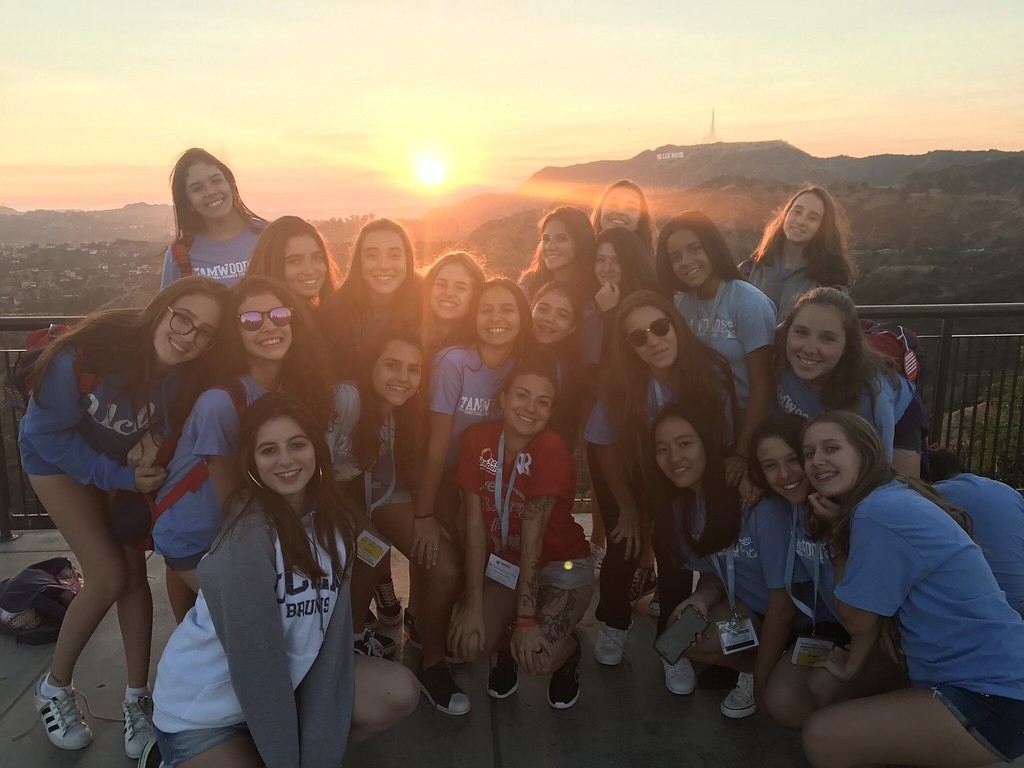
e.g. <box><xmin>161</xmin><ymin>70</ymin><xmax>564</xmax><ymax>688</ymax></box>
<box><xmin>109</xmin><ymin>381</ymin><xmax>249</xmax><ymax>552</ymax></box>
<box><xmin>859</xmin><ymin>318</ymin><xmax>921</xmax><ymax>390</ymax></box>
<box><xmin>12</xmin><ymin>323</ymin><xmax>104</xmax><ymax>409</ymax></box>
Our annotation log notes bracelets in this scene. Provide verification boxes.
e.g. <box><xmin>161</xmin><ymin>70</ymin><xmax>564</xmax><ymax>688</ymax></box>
<box><xmin>515</xmin><ymin>618</ymin><xmax>540</xmax><ymax>627</ymax></box>
<box><xmin>415</xmin><ymin>512</ymin><xmax>435</xmax><ymax>519</ymax></box>
<box><xmin>514</xmin><ymin>614</ymin><xmax>535</xmax><ymax>620</ymax></box>
<box><xmin>431</xmin><ymin>546</ymin><xmax>440</xmax><ymax>551</ymax></box>
<box><xmin>824</xmin><ymin>543</ymin><xmax>844</xmax><ymax>561</ymax></box>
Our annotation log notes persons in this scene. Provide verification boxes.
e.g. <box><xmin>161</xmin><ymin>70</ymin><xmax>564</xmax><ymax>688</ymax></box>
<box><xmin>152</xmin><ymin>393</ymin><xmax>421</xmax><ymax>768</ymax></box>
<box><xmin>18</xmin><ymin>277</ymin><xmax>237</xmax><ymax>759</ymax></box>
<box><xmin>159</xmin><ymin>148</ymin><xmax>272</xmax><ymax>292</ymax></box>
<box><xmin>152</xmin><ymin>179</ymin><xmax>1024</xmax><ymax>731</ymax></box>
<box><xmin>774</xmin><ymin>412</ymin><xmax>1024</xmax><ymax>768</ymax></box>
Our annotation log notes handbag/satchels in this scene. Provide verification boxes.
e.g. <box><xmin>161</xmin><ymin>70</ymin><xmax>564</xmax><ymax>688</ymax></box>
<box><xmin>0</xmin><ymin>557</ymin><xmax>84</xmax><ymax>645</ymax></box>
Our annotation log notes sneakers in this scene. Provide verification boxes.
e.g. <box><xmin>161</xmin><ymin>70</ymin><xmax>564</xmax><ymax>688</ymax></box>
<box><xmin>404</xmin><ymin>608</ymin><xmax>416</xmax><ymax>632</ymax></box>
<box><xmin>413</xmin><ymin>652</ymin><xmax>471</xmax><ymax>715</ymax></box>
<box><xmin>121</xmin><ymin>696</ymin><xmax>154</xmax><ymax>758</ymax></box>
<box><xmin>486</xmin><ymin>649</ymin><xmax>520</xmax><ymax>699</ymax></box>
<box><xmin>12</xmin><ymin>674</ymin><xmax>124</xmax><ymax>750</ymax></box>
<box><xmin>589</xmin><ymin>541</ymin><xmax>607</xmax><ymax>582</ymax></box>
<box><xmin>354</xmin><ymin>632</ymin><xmax>402</xmax><ymax>664</ymax></box>
<box><xmin>547</xmin><ymin>632</ymin><xmax>582</xmax><ymax>709</ymax></box>
<box><xmin>137</xmin><ymin>735</ymin><xmax>162</xmax><ymax>768</ymax></box>
<box><xmin>661</xmin><ymin>656</ymin><xmax>695</xmax><ymax>694</ymax></box>
<box><xmin>628</xmin><ymin>566</ymin><xmax>658</xmax><ymax>609</ymax></box>
<box><xmin>365</xmin><ymin>629</ymin><xmax>396</xmax><ymax>655</ymax></box>
<box><xmin>649</xmin><ymin>590</ymin><xmax>660</xmax><ymax>617</ymax></box>
<box><xmin>408</xmin><ymin>618</ymin><xmax>467</xmax><ymax>664</ymax></box>
<box><xmin>373</xmin><ymin>579</ymin><xmax>403</xmax><ymax>626</ymax></box>
<box><xmin>720</xmin><ymin>672</ymin><xmax>757</xmax><ymax>718</ymax></box>
<box><xmin>593</xmin><ymin>621</ymin><xmax>635</xmax><ymax>665</ymax></box>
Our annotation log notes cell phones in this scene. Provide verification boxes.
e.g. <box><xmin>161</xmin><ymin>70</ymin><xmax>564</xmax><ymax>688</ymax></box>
<box><xmin>653</xmin><ymin>604</ymin><xmax>709</xmax><ymax>666</ymax></box>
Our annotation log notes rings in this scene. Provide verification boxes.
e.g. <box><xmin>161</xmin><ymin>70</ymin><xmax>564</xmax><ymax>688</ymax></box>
<box><xmin>535</xmin><ymin>648</ymin><xmax>543</xmax><ymax>654</ymax></box>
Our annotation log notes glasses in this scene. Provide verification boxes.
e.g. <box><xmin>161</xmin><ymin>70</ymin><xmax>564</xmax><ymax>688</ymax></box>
<box><xmin>167</xmin><ymin>306</ymin><xmax>220</xmax><ymax>352</ymax></box>
<box><xmin>626</xmin><ymin>317</ymin><xmax>672</xmax><ymax>347</ymax></box>
<box><xmin>232</xmin><ymin>306</ymin><xmax>295</xmax><ymax>332</ymax></box>
